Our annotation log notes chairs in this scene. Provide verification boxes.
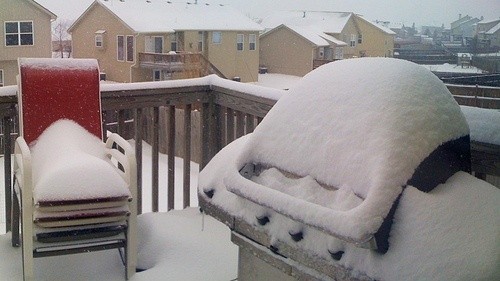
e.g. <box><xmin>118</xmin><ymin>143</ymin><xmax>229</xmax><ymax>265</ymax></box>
<box><xmin>9</xmin><ymin>56</ymin><xmax>136</xmax><ymax>280</ymax></box>
